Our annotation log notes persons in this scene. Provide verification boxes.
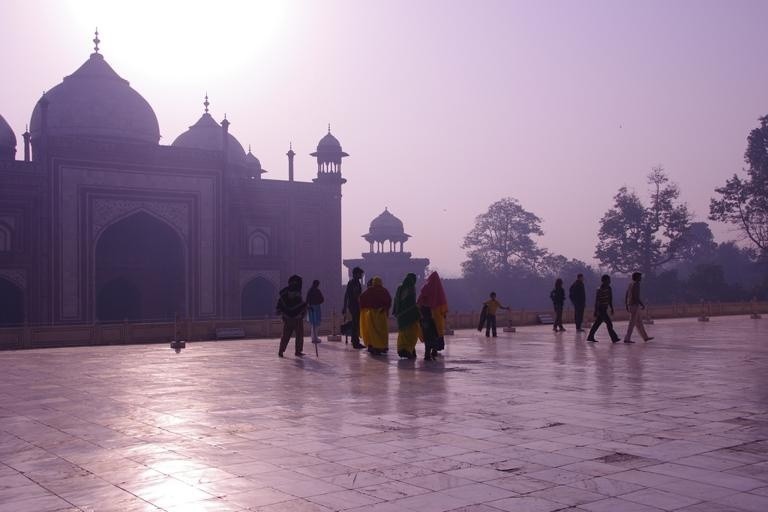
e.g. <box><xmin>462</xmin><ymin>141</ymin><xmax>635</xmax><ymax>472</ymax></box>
<box><xmin>621</xmin><ymin>272</ymin><xmax>655</xmax><ymax>343</ymax></box>
<box><xmin>359</xmin><ymin>277</ymin><xmax>389</xmax><ymax>355</ymax></box>
<box><xmin>416</xmin><ymin>270</ymin><xmax>454</xmax><ymax>361</ymax></box>
<box><xmin>549</xmin><ymin>277</ymin><xmax>566</xmax><ymax>332</ymax></box>
<box><xmin>481</xmin><ymin>290</ymin><xmax>509</xmax><ymax>338</ymax></box>
<box><xmin>341</xmin><ymin>265</ymin><xmax>360</xmax><ymax>349</ymax></box>
<box><xmin>392</xmin><ymin>271</ymin><xmax>417</xmax><ymax>361</ymax></box>
<box><xmin>273</xmin><ymin>271</ymin><xmax>307</xmax><ymax>361</ymax></box>
<box><xmin>305</xmin><ymin>278</ymin><xmax>321</xmax><ymax>344</ymax></box>
<box><xmin>568</xmin><ymin>273</ymin><xmax>587</xmax><ymax>332</ymax></box>
<box><xmin>585</xmin><ymin>273</ymin><xmax>621</xmax><ymax>344</ymax></box>
